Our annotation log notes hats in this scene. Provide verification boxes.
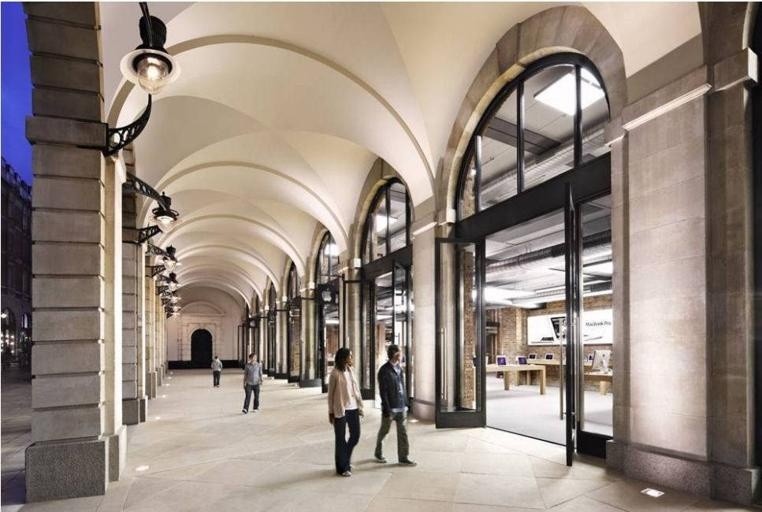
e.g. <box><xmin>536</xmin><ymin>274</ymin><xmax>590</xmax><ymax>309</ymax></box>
<box><xmin>387</xmin><ymin>344</ymin><xmax>400</xmax><ymax>360</ymax></box>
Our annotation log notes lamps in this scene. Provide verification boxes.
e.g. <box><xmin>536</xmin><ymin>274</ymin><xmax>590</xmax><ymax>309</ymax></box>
<box><xmin>103</xmin><ymin>1</ymin><xmax>180</xmax><ymax>156</ymax></box>
<box><xmin>122</xmin><ymin>172</ymin><xmax>181</xmax><ymax>319</ymax></box>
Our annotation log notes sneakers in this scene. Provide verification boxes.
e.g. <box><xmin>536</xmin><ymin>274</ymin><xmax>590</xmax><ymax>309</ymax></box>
<box><xmin>374</xmin><ymin>452</ymin><xmax>387</xmax><ymax>463</ymax></box>
<box><xmin>242</xmin><ymin>408</ymin><xmax>258</xmax><ymax>414</ymax></box>
<box><xmin>398</xmin><ymin>457</ymin><xmax>416</xmax><ymax>466</ymax></box>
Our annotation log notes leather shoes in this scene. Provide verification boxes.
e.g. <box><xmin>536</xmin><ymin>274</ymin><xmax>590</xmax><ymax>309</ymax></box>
<box><xmin>342</xmin><ymin>463</ymin><xmax>353</xmax><ymax>476</ymax></box>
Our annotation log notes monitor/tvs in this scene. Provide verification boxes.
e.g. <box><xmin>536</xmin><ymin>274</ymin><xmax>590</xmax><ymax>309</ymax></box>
<box><xmin>496</xmin><ymin>355</ymin><xmax>507</xmax><ymax>366</ymax></box>
<box><xmin>516</xmin><ymin>356</ymin><xmax>527</xmax><ymax>365</ymax></box>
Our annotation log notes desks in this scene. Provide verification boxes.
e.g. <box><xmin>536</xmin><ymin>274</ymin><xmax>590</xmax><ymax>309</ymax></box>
<box><xmin>485</xmin><ymin>359</ymin><xmax>613</xmax><ymax>395</ymax></box>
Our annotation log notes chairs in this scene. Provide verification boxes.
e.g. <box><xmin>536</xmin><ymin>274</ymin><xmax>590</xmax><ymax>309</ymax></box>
<box><xmin>496</xmin><ymin>352</ymin><xmax>554</xmax><ymax>378</ymax></box>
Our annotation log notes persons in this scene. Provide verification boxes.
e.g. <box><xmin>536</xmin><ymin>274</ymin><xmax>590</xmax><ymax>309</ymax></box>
<box><xmin>210</xmin><ymin>356</ymin><xmax>223</xmax><ymax>388</ymax></box>
<box><xmin>326</xmin><ymin>347</ymin><xmax>365</xmax><ymax>477</ymax></box>
<box><xmin>373</xmin><ymin>344</ymin><xmax>419</xmax><ymax>467</ymax></box>
<box><xmin>240</xmin><ymin>352</ymin><xmax>263</xmax><ymax>414</ymax></box>
